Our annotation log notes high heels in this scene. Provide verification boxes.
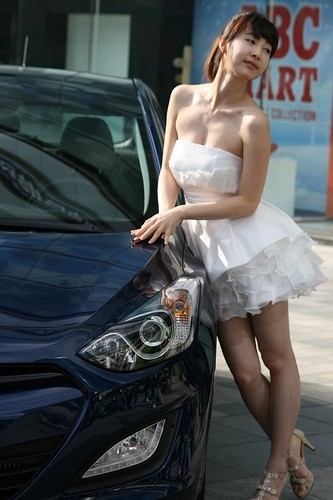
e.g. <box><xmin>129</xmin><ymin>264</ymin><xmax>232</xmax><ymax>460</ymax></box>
<box><xmin>249</xmin><ymin>469</ymin><xmax>288</xmax><ymax>500</ymax></box>
<box><xmin>286</xmin><ymin>430</ymin><xmax>316</xmax><ymax>499</ymax></box>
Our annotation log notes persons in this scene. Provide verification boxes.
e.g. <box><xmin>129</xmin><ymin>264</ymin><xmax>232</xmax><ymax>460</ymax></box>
<box><xmin>129</xmin><ymin>10</ymin><xmax>330</xmax><ymax>500</ymax></box>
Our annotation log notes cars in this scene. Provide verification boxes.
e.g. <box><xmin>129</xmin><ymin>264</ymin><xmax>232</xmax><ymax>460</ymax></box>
<box><xmin>0</xmin><ymin>34</ymin><xmax>217</xmax><ymax>500</ymax></box>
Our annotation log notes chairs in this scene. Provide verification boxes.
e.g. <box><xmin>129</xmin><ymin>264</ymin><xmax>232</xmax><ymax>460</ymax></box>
<box><xmin>1</xmin><ymin>108</ymin><xmax>147</xmax><ymax>222</ymax></box>
